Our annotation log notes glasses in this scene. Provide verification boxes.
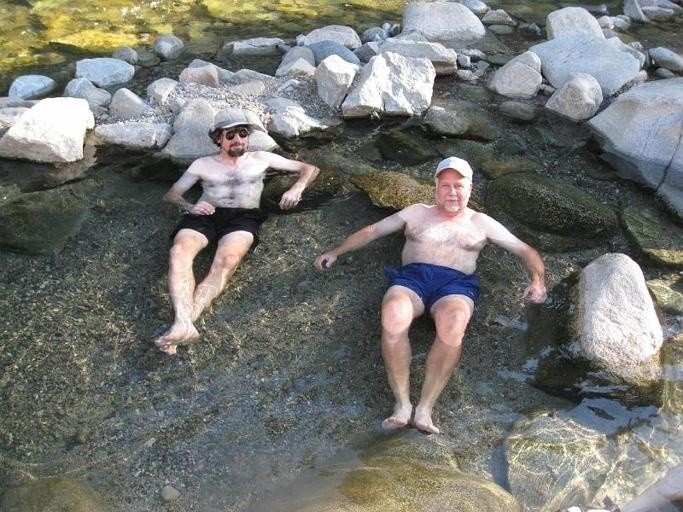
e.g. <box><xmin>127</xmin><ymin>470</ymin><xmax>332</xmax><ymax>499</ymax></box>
<box><xmin>226</xmin><ymin>129</ymin><xmax>248</xmax><ymax>140</ymax></box>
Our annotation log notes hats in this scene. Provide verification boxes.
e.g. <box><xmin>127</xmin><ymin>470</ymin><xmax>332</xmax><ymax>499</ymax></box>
<box><xmin>435</xmin><ymin>157</ymin><xmax>474</xmax><ymax>178</ymax></box>
<box><xmin>214</xmin><ymin>108</ymin><xmax>250</xmax><ymax>130</ymax></box>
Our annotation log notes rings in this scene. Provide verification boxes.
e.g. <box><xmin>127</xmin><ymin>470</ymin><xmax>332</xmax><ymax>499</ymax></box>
<box><xmin>199</xmin><ymin>208</ymin><xmax>204</xmax><ymax>212</ymax></box>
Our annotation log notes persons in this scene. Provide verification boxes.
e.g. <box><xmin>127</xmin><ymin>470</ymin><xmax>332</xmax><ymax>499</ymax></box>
<box><xmin>313</xmin><ymin>156</ymin><xmax>546</xmax><ymax>433</ymax></box>
<box><xmin>153</xmin><ymin>107</ymin><xmax>320</xmax><ymax>358</ymax></box>
